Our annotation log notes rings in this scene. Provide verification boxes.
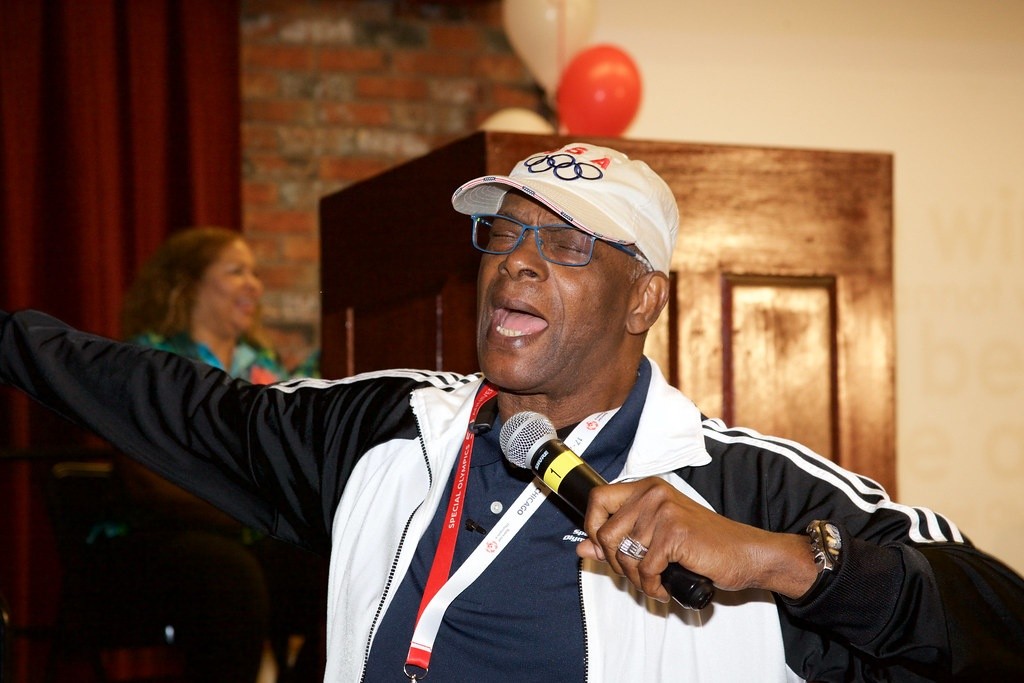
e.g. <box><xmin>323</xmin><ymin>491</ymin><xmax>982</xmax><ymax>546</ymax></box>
<box><xmin>614</xmin><ymin>533</ymin><xmax>649</xmax><ymax>565</ymax></box>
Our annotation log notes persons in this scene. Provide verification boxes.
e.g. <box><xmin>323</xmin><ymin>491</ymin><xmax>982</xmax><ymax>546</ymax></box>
<box><xmin>0</xmin><ymin>144</ymin><xmax>1024</xmax><ymax>683</ymax></box>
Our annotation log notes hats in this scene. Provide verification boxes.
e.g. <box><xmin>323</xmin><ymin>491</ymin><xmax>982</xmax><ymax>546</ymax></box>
<box><xmin>452</xmin><ymin>143</ymin><xmax>680</xmax><ymax>278</ymax></box>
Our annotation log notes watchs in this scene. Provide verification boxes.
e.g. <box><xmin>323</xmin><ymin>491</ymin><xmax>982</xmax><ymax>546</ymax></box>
<box><xmin>790</xmin><ymin>520</ymin><xmax>842</xmax><ymax>602</ymax></box>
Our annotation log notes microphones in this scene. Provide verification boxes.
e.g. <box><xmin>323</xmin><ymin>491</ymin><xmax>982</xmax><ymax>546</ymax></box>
<box><xmin>499</xmin><ymin>412</ymin><xmax>715</xmax><ymax>610</ymax></box>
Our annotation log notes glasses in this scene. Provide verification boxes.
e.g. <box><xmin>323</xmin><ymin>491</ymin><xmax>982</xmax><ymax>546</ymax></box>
<box><xmin>470</xmin><ymin>213</ymin><xmax>654</xmax><ymax>272</ymax></box>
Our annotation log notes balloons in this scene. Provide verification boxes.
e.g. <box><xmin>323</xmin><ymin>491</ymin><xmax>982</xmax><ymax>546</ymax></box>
<box><xmin>554</xmin><ymin>46</ymin><xmax>644</xmax><ymax>138</ymax></box>
<box><xmin>482</xmin><ymin>109</ymin><xmax>557</xmax><ymax>136</ymax></box>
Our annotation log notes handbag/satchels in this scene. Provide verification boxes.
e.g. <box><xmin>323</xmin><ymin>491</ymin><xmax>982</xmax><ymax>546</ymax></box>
<box><xmin>42</xmin><ymin>468</ymin><xmax>293</xmax><ymax>645</ymax></box>
